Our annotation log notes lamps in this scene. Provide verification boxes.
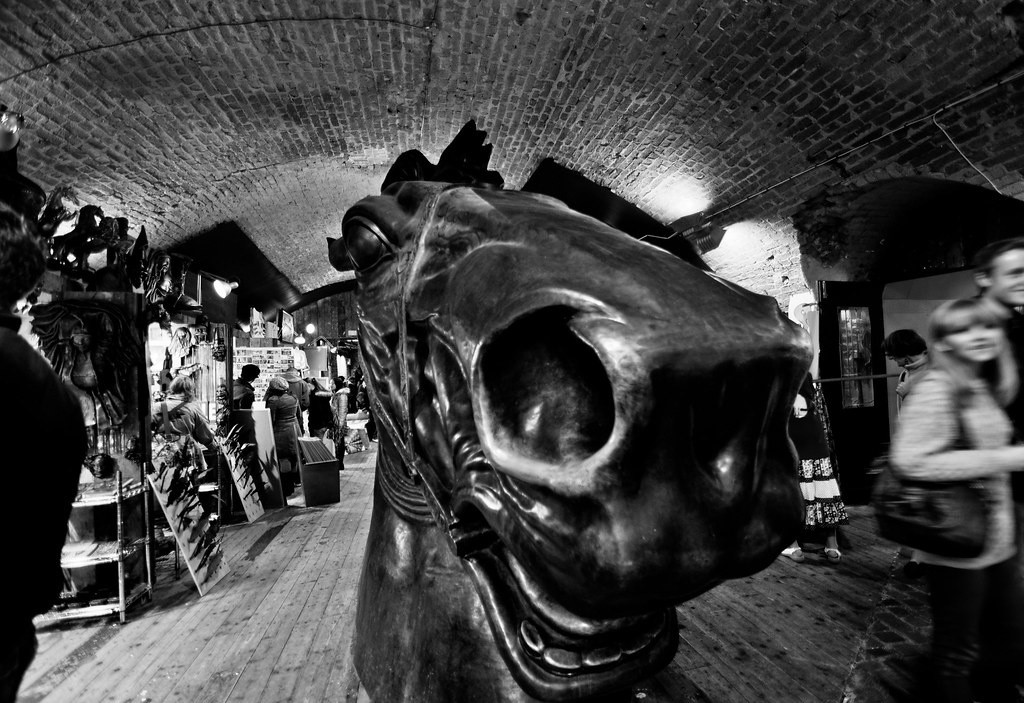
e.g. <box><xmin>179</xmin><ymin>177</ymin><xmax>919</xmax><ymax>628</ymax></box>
<box><xmin>294</xmin><ymin>324</ymin><xmax>316</xmax><ymax>345</ymax></box>
<box><xmin>694</xmin><ymin>211</ymin><xmax>726</xmax><ymax>255</ymax></box>
<box><xmin>212</xmin><ymin>278</ymin><xmax>240</xmax><ymax>299</ymax></box>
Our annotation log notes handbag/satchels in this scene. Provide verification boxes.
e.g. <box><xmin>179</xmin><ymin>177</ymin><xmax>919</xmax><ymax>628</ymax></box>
<box><xmin>875</xmin><ymin>368</ymin><xmax>994</xmax><ymax>560</ymax></box>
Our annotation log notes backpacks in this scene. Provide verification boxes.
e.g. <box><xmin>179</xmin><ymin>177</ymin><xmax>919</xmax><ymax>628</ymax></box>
<box><xmin>355</xmin><ymin>384</ymin><xmax>365</xmax><ymax>409</ymax></box>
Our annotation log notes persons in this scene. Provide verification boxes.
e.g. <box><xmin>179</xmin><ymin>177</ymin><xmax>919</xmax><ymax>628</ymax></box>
<box><xmin>283</xmin><ymin>367</ymin><xmax>309</xmax><ymax>487</ymax></box>
<box><xmin>779</xmin><ymin>371</ymin><xmax>849</xmax><ymax>563</ymax></box>
<box><xmin>266</xmin><ymin>377</ymin><xmax>301</xmax><ymax>494</ymax></box>
<box><xmin>302</xmin><ymin>376</ymin><xmax>334</xmax><ymax>438</ymax></box>
<box><xmin>234</xmin><ymin>364</ymin><xmax>260</xmax><ymax>409</ymax></box>
<box><xmin>329</xmin><ymin>377</ymin><xmax>350</xmax><ymax>470</ymax></box>
<box><xmin>339</xmin><ymin>376</ymin><xmax>370</xmax><ymax>413</ymax></box>
<box><xmin>169</xmin><ymin>374</ymin><xmax>213</xmax><ymax>446</ymax></box>
<box><xmin>0</xmin><ymin>203</ymin><xmax>88</xmax><ymax>703</ymax></box>
<box><xmin>888</xmin><ymin>236</ymin><xmax>1024</xmax><ymax>703</ymax></box>
<box><xmin>886</xmin><ymin>328</ymin><xmax>933</xmax><ymax>591</ymax></box>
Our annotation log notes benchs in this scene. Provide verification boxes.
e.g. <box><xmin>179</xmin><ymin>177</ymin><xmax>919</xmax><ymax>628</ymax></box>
<box><xmin>292</xmin><ymin>422</ymin><xmax>340</xmax><ymax>508</ymax></box>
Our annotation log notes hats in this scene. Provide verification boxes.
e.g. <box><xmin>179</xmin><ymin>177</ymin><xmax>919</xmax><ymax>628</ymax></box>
<box><xmin>270</xmin><ymin>376</ymin><xmax>289</xmax><ymax>390</ymax></box>
<box><xmin>285</xmin><ymin>368</ymin><xmax>301</xmax><ymax>382</ymax></box>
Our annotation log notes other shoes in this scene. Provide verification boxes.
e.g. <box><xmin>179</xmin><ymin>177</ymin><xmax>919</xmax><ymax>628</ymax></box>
<box><xmin>294</xmin><ymin>483</ymin><xmax>301</xmax><ymax>486</ymax></box>
<box><xmin>372</xmin><ymin>438</ymin><xmax>378</xmax><ymax>442</ymax></box>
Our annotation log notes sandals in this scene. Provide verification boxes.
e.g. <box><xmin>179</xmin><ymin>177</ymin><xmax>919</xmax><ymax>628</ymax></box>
<box><xmin>825</xmin><ymin>546</ymin><xmax>841</xmax><ymax>560</ymax></box>
<box><xmin>781</xmin><ymin>548</ymin><xmax>804</xmax><ymax>562</ymax></box>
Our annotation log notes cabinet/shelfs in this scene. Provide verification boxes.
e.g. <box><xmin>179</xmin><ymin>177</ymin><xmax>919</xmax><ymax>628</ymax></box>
<box><xmin>198</xmin><ymin>436</ymin><xmax>221</xmax><ymax>527</ymax></box>
<box><xmin>35</xmin><ymin>464</ymin><xmax>151</xmax><ymax>630</ymax></box>
